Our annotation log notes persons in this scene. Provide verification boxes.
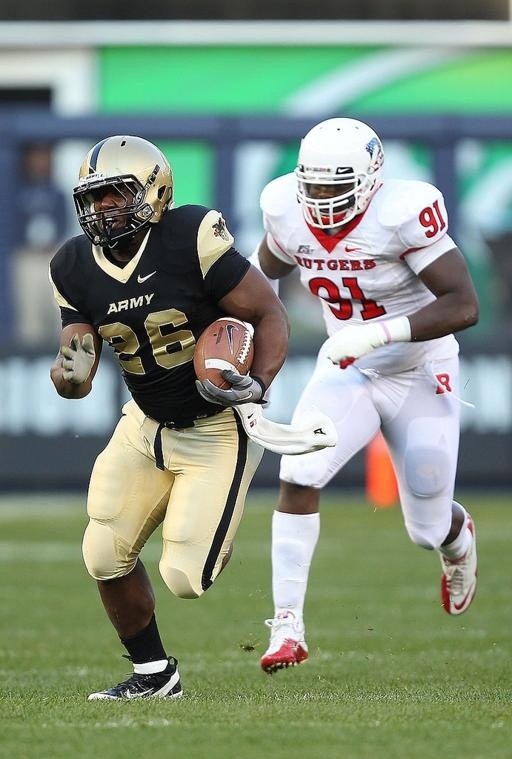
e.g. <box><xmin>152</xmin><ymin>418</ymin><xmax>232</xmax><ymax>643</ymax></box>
<box><xmin>47</xmin><ymin>135</ymin><xmax>338</xmax><ymax>701</ymax></box>
<box><xmin>242</xmin><ymin>117</ymin><xmax>481</xmax><ymax>674</ymax></box>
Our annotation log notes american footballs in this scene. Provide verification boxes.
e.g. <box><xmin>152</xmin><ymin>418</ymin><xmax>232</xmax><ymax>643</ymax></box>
<box><xmin>193</xmin><ymin>316</ymin><xmax>254</xmax><ymax>391</ymax></box>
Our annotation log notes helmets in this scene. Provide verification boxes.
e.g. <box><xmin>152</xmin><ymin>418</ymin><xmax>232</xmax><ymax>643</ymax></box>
<box><xmin>72</xmin><ymin>135</ymin><xmax>173</xmax><ymax>246</ymax></box>
<box><xmin>294</xmin><ymin>118</ymin><xmax>384</xmax><ymax>229</ymax></box>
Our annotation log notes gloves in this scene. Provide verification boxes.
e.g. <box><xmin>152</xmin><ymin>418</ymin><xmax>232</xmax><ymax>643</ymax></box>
<box><xmin>60</xmin><ymin>334</ymin><xmax>96</xmax><ymax>384</ymax></box>
<box><xmin>195</xmin><ymin>369</ymin><xmax>263</xmax><ymax>406</ymax></box>
<box><xmin>323</xmin><ymin>316</ymin><xmax>411</xmax><ymax>369</ymax></box>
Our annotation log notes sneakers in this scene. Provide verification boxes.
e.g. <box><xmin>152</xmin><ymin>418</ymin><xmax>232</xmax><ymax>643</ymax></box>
<box><xmin>441</xmin><ymin>513</ymin><xmax>477</xmax><ymax>615</ymax></box>
<box><xmin>87</xmin><ymin>656</ymin><xmax>183</xmax><ymax>700</ymax></box>
<box><xmin>261</xmin><ymin>609</ymin><xmax>309</xmax><ymax>673</ymax></box>
